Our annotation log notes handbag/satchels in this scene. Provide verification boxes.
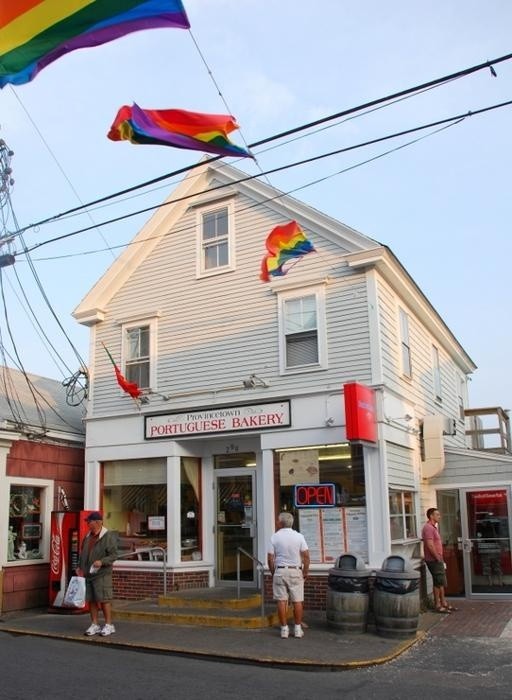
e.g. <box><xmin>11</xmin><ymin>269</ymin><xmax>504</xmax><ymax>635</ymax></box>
<box><xmin>64</xmin><ymin>576</ymin><xmax>86</xmax><ymax>608</ymax></box>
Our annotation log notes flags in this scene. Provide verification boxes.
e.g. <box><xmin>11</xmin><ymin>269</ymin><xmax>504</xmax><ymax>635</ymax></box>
<box><xmin>0</xmin><ymin>0</ymin><xmax>193</xmax><ymax>92</ymax></box>
<box><xmin>259</xmin><ymin>217</ymin><xmax>316</xmax><ymax>281</ymax></box>
<box><xmin>106</xmin><ymin>102</ymin><xmax>254</xmax><ymax>165</ymax></box>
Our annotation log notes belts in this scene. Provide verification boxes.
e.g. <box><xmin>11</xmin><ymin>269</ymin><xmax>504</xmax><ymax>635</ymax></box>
<box><xmin>278</xmin><ymin>566</ymin><xmax>302</xmax><ymax>569</ymax></box>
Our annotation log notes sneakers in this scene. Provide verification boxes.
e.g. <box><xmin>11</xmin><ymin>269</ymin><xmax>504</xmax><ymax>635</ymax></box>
<box><xmin>85</xmin><ymin>623</ymin><xmax>116</xmax><ymax>636</ymax></box>
<box><xmin>280</xmin><ymin>624</ymin><xmax>305</xmax><ymax>639</ymax></box>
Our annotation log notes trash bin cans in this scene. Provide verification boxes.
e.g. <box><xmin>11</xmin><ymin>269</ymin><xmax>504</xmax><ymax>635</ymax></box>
<box><xmin>325</xmin><ymin>554</ymin><xmax>423</xmax><ymax>640</ymax></box>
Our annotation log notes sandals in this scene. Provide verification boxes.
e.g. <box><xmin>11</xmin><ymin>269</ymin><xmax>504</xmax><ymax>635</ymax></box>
<box><xmin>436</xmin><ymin>600</ymin><xmax>454</xmax><ymax>613</ymax></box>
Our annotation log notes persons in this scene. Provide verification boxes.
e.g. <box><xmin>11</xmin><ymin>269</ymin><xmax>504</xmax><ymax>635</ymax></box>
<box><xmin>268</xmin><ymin>512</ymin><xmax>310</xmax><ymax>638</ymax></box>
<box><xmin>477</xmin><ymin>509</ymin><xmax>504</xmax><ymax>587</ymax></box>
<box><xmin>74</xmin><ymin>511</ymin><xmax>117</xmax><ymax>636</ymax></box>
<box><xmin>421</xmin><ymin>508</ymin><xmax>458</xmax><ymax>612</ymax></box>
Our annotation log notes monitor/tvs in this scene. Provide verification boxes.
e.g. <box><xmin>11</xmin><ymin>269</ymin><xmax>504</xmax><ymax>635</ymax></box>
<box><xmin>145</xmin><ymin>513</ymin><xmax>167</xmax><ymax>536</ymax></box>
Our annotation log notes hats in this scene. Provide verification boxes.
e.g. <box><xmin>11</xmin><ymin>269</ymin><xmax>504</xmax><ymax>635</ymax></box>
<box><xmin>85</xmin><ymin>512</ymin><xmax>102</xmax><ymax>520</ymax></box>
